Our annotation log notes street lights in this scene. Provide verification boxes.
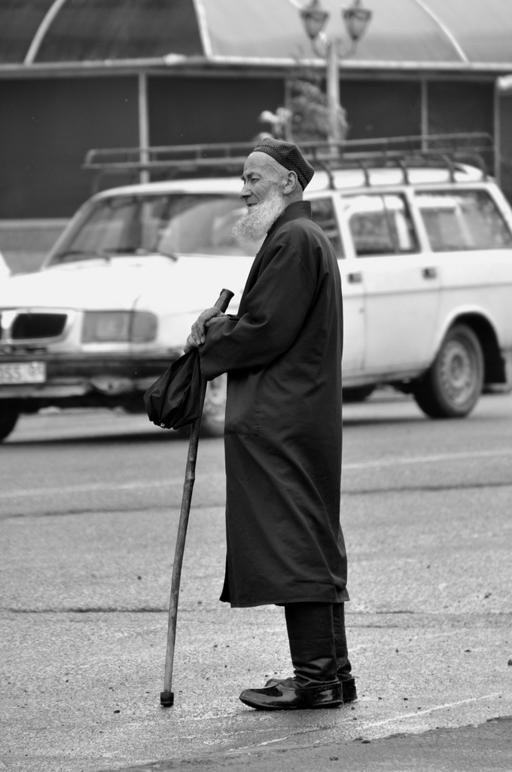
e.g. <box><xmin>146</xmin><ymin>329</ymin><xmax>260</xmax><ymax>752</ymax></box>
<box><xmin>300</xmin><ymin>1</ymin><xmax>371</xmax><ymax>153</ymax></box>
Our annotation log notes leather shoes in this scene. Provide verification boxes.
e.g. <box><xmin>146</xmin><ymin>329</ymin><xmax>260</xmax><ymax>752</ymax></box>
<box><xmin>239</xmin><ymin>675</ymin><xmax>357</xmax><ymax>713</ymax></box>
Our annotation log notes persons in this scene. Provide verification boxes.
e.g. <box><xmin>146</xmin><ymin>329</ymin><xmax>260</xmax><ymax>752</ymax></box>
<box><xmin>184</xmin><ymin>137</ymin><xmax>358</xmax><ymax>711</ymax></box>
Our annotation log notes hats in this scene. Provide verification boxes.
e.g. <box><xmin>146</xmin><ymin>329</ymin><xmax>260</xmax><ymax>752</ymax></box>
<box><xmin>253</xmin><ymin>138</ymin><xmax>314</xmax><ymax>191</ymax></box>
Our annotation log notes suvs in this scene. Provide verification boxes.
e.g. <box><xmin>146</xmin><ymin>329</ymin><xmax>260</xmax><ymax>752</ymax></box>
<box><xmin>0</xmin><ymin>130</ymin><xmax>510</xmax><ymax>438</ymax></box>
